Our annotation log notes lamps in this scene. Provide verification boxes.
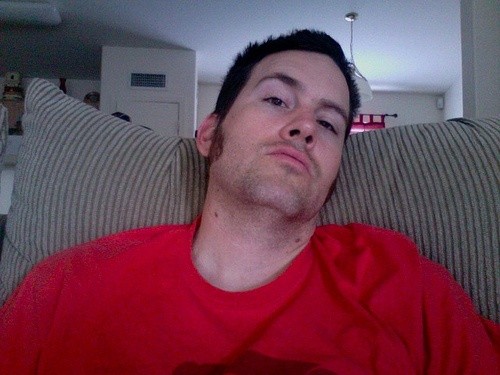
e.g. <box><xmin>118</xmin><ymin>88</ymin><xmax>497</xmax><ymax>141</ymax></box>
<box><xmin>343</xmin><ymin>12</ymin><xmax>374</xmax><ymax>108</ymax></box>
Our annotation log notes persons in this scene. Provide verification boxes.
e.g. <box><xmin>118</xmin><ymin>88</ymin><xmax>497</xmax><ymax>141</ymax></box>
<box><xmin>0</xmin><ymin>27</ymin><xmax>499</xmax><ymax>374</ymax></box>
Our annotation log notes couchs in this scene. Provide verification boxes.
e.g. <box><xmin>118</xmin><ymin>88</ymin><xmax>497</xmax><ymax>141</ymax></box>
<box><xmin>1</xmin><ymin>73</ymin><xmax>500</xmax><ymax>375</ymax></box>
